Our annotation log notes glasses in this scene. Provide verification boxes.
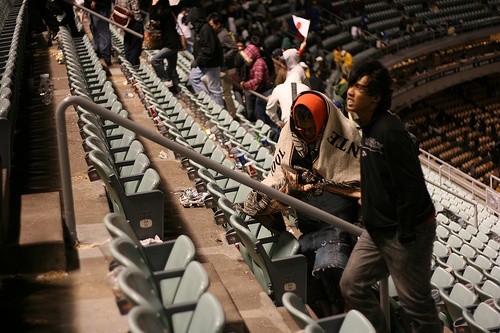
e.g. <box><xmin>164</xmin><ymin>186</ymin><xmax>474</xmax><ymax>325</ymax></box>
<box><xmin>294</xmin><ymin>124</ymin><xmax>316</xmax><ymax>134</ymax></box>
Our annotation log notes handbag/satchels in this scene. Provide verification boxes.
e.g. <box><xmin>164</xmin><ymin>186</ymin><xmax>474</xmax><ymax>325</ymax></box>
<box><xmin>109</xmin><ymin>4</ymin><xmax>134</xmax><ymax>27</ymax></box>
<box><xmin>177</xmin><ymin>34</ymin><xmax>188</xmax><ymax>51</ymax></box>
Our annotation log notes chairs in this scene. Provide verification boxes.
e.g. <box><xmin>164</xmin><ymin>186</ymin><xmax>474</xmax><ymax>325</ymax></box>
<box><xmin>57</xmin><ymin>10</ymin><xmax>500</xmax><ymax>333</ymax></box>
<box><xmin>0</xmin><ymin>0</ymin><xmax>28</xmax><ymax>240</ymax></box>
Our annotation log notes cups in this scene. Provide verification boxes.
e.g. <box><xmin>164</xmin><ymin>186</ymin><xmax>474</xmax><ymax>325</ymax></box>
<box><xmin>40</xmin><ymin>73</ymin><xmax>50</xmax><ymax>91</ymax></box>
<box><xmin>43</xmin><ymin>89</ymin><xmax>53</xmax><ymax>106</ymax></box>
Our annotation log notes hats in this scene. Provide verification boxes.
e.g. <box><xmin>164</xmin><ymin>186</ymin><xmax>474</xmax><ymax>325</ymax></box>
<box><xmin>299</xmin><ymin>62</ymin><xmax>309</xmax><ymax>69</ymax></box>
<box><xmin>272</xmin><ymin>49</ymin><xmax>285</xmax><ymax>60</ymax></box>
<box><xmin>315</xmin><ymin>56</ymin><xmax>323</xmax><ymax>61</ymax></box>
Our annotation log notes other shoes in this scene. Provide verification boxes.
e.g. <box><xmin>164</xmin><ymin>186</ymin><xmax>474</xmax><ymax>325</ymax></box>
<box><xmin>56</xmin><ymin>50</ymin><xmax>66</xmax><ymax>63</ymax></box>
<box><xmin>94</xmin><ymin>49</ymin><xmax>112</xmax><ymax>65</ymax></box>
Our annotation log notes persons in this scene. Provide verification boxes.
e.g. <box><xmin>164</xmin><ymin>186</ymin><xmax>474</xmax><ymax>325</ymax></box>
<box><xmin>344</xmin><ymin>60</ymin><xmax>444</xmax><ymax>333</ymax></box>
<box><xmin>233</xmin><ymin>91</ymin><xmax>367</xmax><ymax>317</ymax></box>
<box><xmin>266</xmin><ymin>65</ymin><xmax>311</xmax><ymax>128</ymax></box>
<box><xmin>31</xmin><ymin>0</ymin><xmax>390</xmax><ymax>123</ymax></box>
<box><xmin>408</xmin><ymin>97</ymin><xmax>500</xmax><ymax>190</ymax></box>
<box><xmin>393</xmin><ymin>0</ymin><xmax>500</xmax><ymax>80</ymax></box>
